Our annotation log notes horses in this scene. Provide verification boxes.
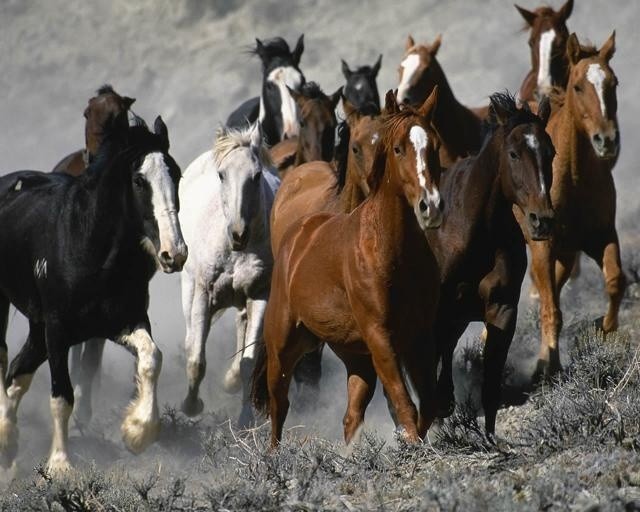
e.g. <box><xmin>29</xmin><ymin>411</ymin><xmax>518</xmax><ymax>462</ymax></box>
<box><xmin>177</xmin><ymin>1</ymin><xmax>626</xmax><ymax>458</ymax></box>
<box><xmin>1</xmin><ymin>79</ymin><xmax>188</xmax><ymax>489</ymax></box>
